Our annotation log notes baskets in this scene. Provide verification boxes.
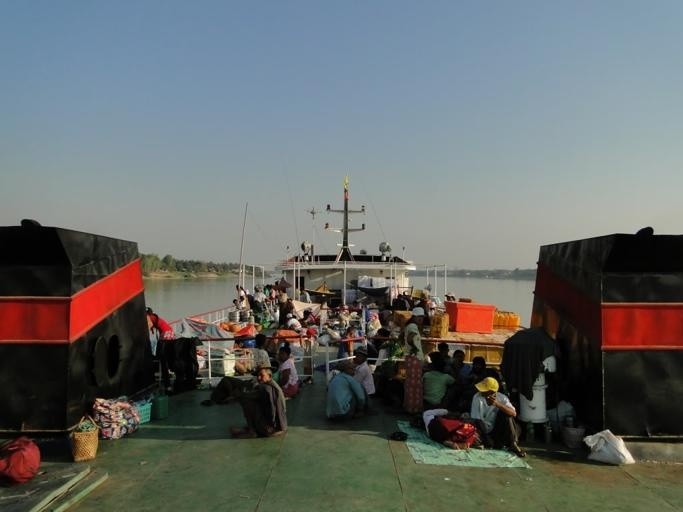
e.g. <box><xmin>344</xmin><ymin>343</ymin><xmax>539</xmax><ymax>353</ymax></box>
<box><xmin>69</xmin><ymin>416</ymin><xmax>102</xmax><ymax>462</ymax></box>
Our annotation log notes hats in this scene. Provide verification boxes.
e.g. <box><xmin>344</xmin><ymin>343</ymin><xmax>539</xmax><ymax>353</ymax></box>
<box><xmin>474</xmin><ymin>376</ymin><xmax>499</xmax><ymax>392</ymax></box>
<box><xmin>412</xmin><ymin>307</ymin><xmax>425</xmax><ymax>317</ymax></box>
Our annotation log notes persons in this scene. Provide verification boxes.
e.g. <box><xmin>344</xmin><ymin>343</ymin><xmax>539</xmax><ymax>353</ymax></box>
<box><xmin>146</xmin><ymin>306</ymin><xmax>177</xmax><ymax>360</ymax></box>
<box><xmin>210</xmin><ymin>280</ymin><xmax>319</xmax><ymax>439</ymax></box>
<box><xmin>445</xmin><ymin>349</ymin><xmax>476</xmax><ymax>385</ymax></box>
<box><xmin>325</xmin><ymin>294</ymin><xmax>463</xmax><ymax>441</ymax></box>
<box><xmin>452</xmin><ymin>356</ymin><xmax>504</xmax><ymax>412</ymax></box>
<box><xmin>417</xmin><ymin>403</ymin><xmax>477</xmax><ymax>451</ymax></box>
<box><xmin>469</xmin><ymin>376</ymin><xmax>526</xmax><ymax>458</ymax></box>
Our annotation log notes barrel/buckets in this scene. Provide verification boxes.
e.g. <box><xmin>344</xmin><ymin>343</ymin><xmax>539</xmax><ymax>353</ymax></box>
<box><xmin>520</xmin><ymin>373</ymin><xmax>547</xmax><ymax>421</ymax></box>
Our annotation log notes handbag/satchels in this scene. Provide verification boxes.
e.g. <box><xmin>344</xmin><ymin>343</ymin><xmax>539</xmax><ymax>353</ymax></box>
<box><xmin>429</xmin><ymin>414</ymin><xmax>481</xmax><ymax>450</ymax></box>
<box><xmin>0</xmin><ymin>436</ymin><xmax>40</xmax><ymax>485</ymax></box>
